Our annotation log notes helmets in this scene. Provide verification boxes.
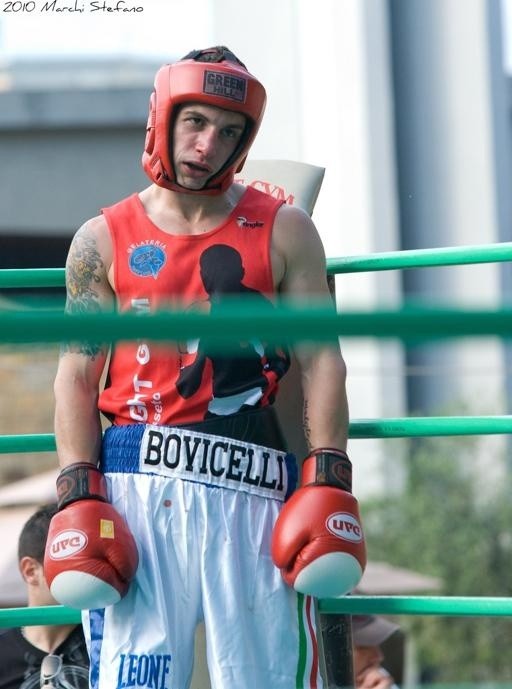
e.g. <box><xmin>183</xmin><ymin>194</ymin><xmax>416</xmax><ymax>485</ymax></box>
<box><xmin>141</xmin><ymin>45</ymin><xmax>268</xmax><ymax>197</ymax></box>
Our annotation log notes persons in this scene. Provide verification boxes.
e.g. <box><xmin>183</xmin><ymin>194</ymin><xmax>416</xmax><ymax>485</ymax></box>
<box><xmin>44</xmin><ymin>40</ymin><xmax>368</xmax><ymax>689</ymax></box>
<box><xmin>349</xmin><ymin>590</ymin><xmax>401</xmax><ymax>689</ymax></box>
<box><xmin>0</xmin><ymin>504</ymin><xmax>98</xmax><ymax>689</ymax></box>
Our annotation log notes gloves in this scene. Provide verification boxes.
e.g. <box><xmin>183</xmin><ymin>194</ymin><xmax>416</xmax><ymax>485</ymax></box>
<box><xmin>43</xmin><ymin>461</ymin><xmax>139</xmax><ymax>609</ymax></box>
<box><xmin>270</xmin><ymin>447</ymin><xmax>367</xmax><ymax>597</ymax></box>
